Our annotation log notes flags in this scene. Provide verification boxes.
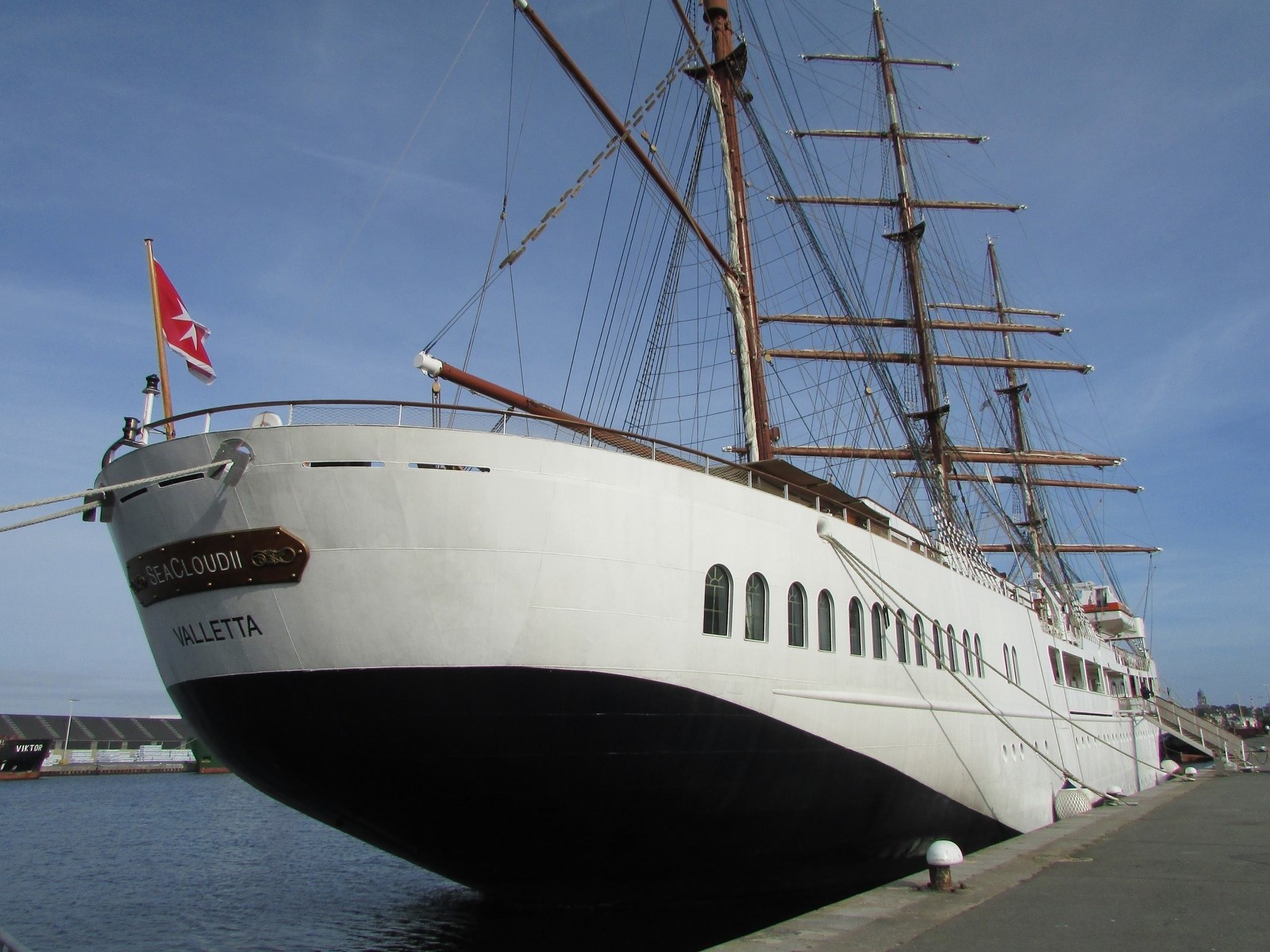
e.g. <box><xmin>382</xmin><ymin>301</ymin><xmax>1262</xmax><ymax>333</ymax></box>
<box><xmin>150</xmin><ymin>254</ymin><xmax>218</xmax><ymax>386</ymax></box>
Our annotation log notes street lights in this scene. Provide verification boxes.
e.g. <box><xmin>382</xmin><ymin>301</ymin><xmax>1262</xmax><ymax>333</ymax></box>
<box><xmin>63</xmin><ymin>699</ymin><xmax>80</xmax><ymax>763</ymax></box>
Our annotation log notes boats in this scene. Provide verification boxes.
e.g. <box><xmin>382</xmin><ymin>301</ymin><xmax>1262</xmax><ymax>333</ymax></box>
<box><xmin>0</xmin><ymin>738</ymin><xmax>54</xmax><ymax>780</ymax></box>
<box><xmin>84</xmin><ymin>1</ymin><xmax>1246</xmax><ymax>930</ymax></box>
